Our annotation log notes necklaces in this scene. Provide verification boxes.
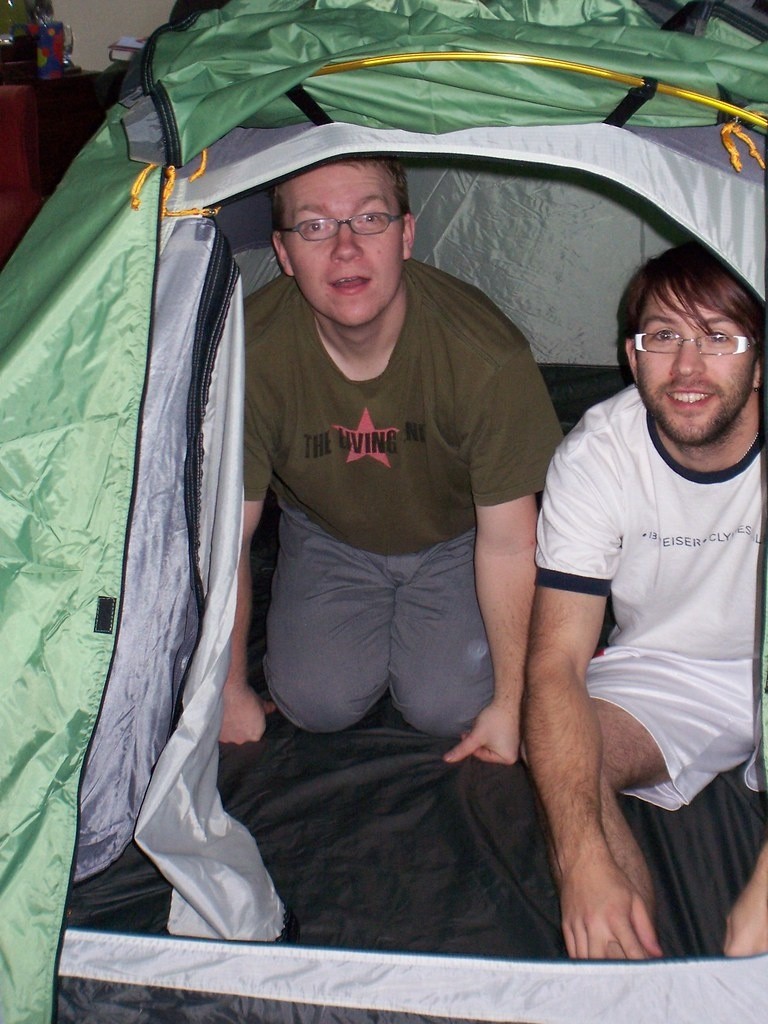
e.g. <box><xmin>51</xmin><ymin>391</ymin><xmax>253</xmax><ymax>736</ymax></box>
<box><xmin>737</xmin><ymin>432</ymin><xmax>757</xmax><ymax>463</ymax></box>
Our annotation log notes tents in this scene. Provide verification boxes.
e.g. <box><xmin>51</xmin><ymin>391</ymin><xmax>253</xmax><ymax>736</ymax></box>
<box><xmin>0</xmin><ymin>0</ymin><xmax>768</xmax><ymax>1024</ymax></box>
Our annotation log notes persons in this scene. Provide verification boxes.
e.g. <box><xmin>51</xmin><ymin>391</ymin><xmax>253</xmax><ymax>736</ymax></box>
<box><xmin>219</xmin><ymin>149</ymin><xmax>564</xmax><ymax>765</ymax></box>
<box><xmin>523</xmin><ymin>233</ymin><xmax>768</xmax><ymax>965</ymax></box>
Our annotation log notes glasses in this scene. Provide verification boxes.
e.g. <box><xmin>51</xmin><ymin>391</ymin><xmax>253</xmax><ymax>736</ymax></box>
<box><xmin>635</xmin><ymin>333</ymin><xmax>749</xmax><ymax>355</ymax></box>
<box><xmin>292</xmin><ymin>212</ymin><xmax>395</xmax><ymax>241</ymax></box>
